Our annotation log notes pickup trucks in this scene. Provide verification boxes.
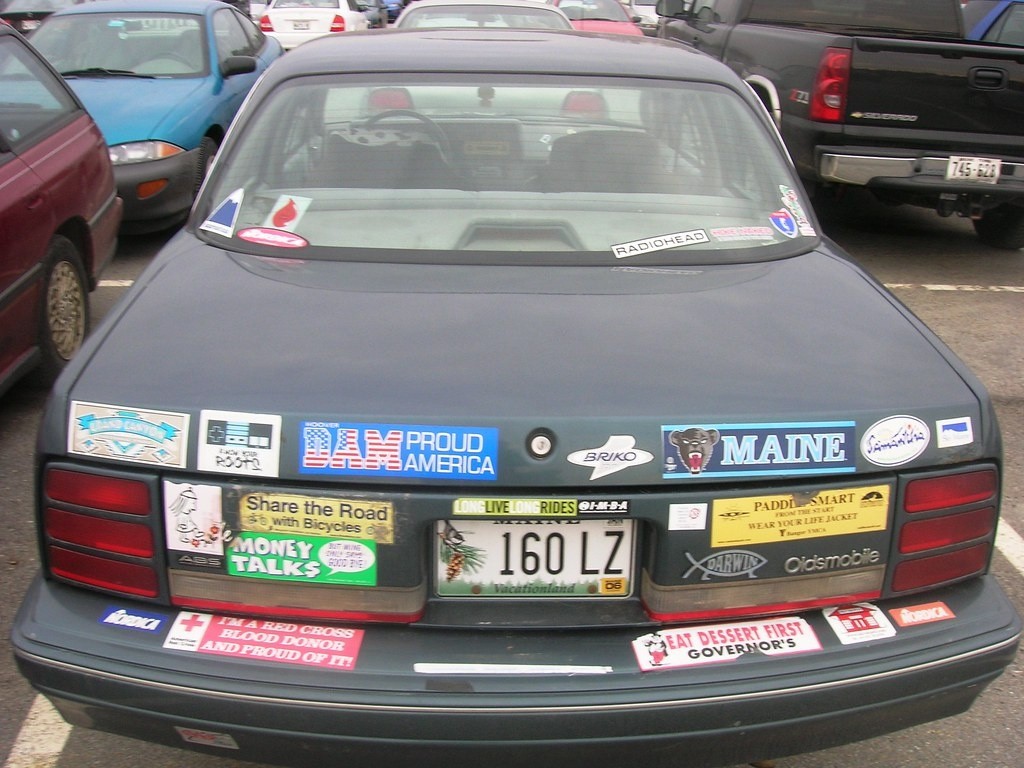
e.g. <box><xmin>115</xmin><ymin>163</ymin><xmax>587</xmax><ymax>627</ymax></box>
<box><xmin>640</xmin><ymin>1</ymin><xmax>1024</xmax><ymax>252</ymax></box>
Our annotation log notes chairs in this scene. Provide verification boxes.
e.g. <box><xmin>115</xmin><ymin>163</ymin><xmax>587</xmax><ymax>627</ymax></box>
<box><xmin>172</xmin><ymin>30</ymin><xmax>202</xmax><ymax>66</ymax></box>
<box><xmin>325</xmin><ymin>129</ymin><xmax>451</xmax><ymax>190</ymax></box>
<box><xmin>544</xmin><ymin>132</ymin><xmax>686</xmax><ymax>195</ymax></box>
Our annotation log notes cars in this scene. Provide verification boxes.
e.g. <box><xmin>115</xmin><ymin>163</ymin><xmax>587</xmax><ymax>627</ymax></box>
<box><xmin>383</xmin><ymin>0</ymin><xmax>404</xmax><ymax>23</ymax></box>
<box><xmin>559</xmin><ymin>1</ymin><xmax>662</xmax><ymax>36</ymax></box>
<box><xmin>322</xmin><ymin>1</ymin><xmax>644</xmax><ymax>164</ymax></box>
<box><xmin>0</xmin><ymin>16</ymin><xmax>125</xmax><ymax>397</ymax></box>
<box><xmin>0</xmin><ymin>1</ymin><xmax>285</xmax><ymax>234</ymax></box>
<box><xmin>259</xmin><ymin>0</ymin><xmax>369</xmax><ymax>51</ymax></box>
<box><xmin>547</xmin><ymin>0</ymin><xmax>644</xmax><ymax>37</ymax></box>
<box><xmin>9</xmin><ymin>27</ymin><xmax>1023</xmax><ymax>768</ymax></box>
<box><xmin>357</xmin><ymin>0</ymin><xmax>389</xmax><ymax>29</ymax></box>
<box><xmin>959</xmin><ymin>0</ymin><xmax>1024</xmax><ymax>46</ymax></box>
